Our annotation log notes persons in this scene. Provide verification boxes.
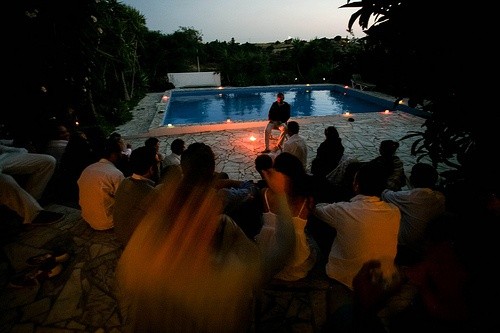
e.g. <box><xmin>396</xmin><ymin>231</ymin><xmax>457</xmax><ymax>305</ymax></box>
<box><xmin>1</xmin><ymin>117</ymin><xmax>499</xmax><ymax>310</ymax></box>
<box><xmin>261</xmin><ymin>93</ymin><xmax>291</xmax><ymax>153</ymax></box>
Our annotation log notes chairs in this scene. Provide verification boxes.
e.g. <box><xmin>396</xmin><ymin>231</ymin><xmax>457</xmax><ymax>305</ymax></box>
<box><xmin>269</xmin><ymin>121</ymin><xmax>288</xmax><ymax>145</ymax></box>
<box><xmin>350</xmin><ymin>74</ymin><xmax>376</xmax><ymax>91</ymax></box>
